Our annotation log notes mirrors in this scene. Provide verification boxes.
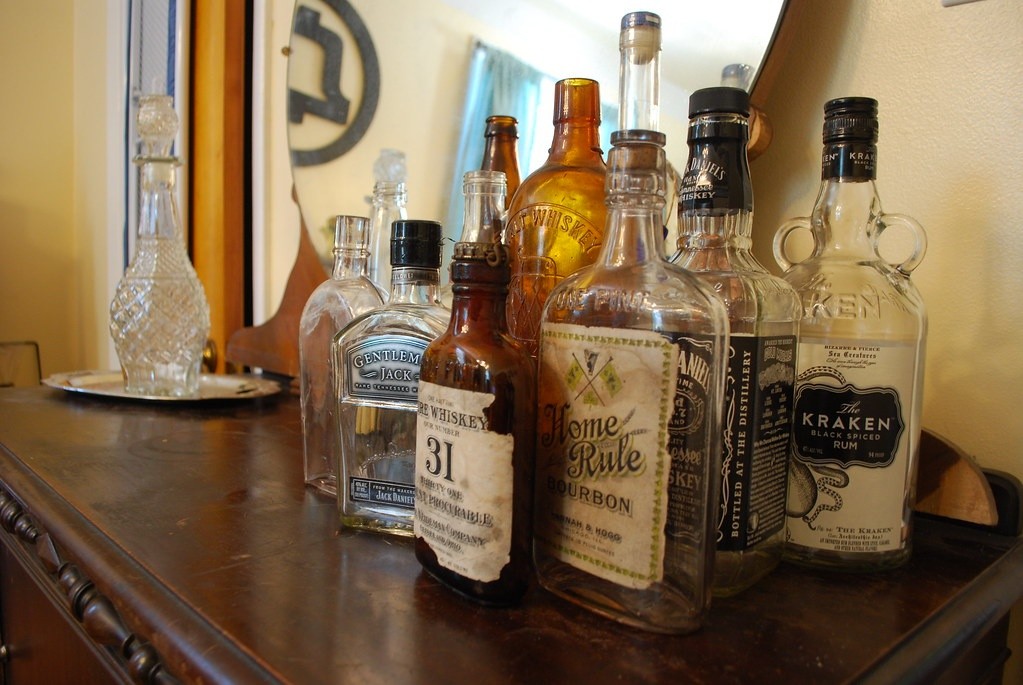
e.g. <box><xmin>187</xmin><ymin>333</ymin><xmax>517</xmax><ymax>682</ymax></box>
<box><xmin>221</xmin><ymin>0</ymin><xmax>789</xmax><ymax>380</ymax></box>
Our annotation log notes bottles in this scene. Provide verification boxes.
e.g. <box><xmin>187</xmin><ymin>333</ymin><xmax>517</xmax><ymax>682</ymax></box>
<box><xmin>109</xmin><ymin>95</ymin><xmax>209</xmax><ymax>398</ymax></box>
<box><xmin>664</xmin><ymin>87</ymin><xmax>801</xmax><ymax>596</ymax></box>
<box><xmin>413</xmin><ymin>242</ymin><xmax>536</xmax><ymax>608</ymax></box>
<box><xmin>619</xmin><ymin>11</ymin><xmax>683</xmax><ymax>260</ymax></box>
<box><xmin>504</xmin><ymin>78</ymin><xmax>607</xmax><ymax>362</ymax></box>
<box><xmin>481</xmin><ymin>116</ymin><xmax>520</xmax><ymax>212</ymax></box>
<box><xmin>368</xmin><ymin>181</ymin><xmax>410</xmax><ymax>293</ymax></box>
<box><xmin>721</xmin><ymin>64</ymin><xmax>756</xmax><ymax>92</ymax></box>
<box><xmin>534</xmin><ymin>130</ymin><xmax>730</xmax><ymax>634</ymax></box>
<box><xmin>440</xmin><ymin>169</ymin><xmax>508</xmax><ymax>310</ymax></box>
<box><xmin>773</xmin><ymin>97</ymin><xmax>927</xmax><ymax>574</ymax></box>
<box><xmin>331</xmin><ymin>220</ymin><xmax>452</xmax><ymax>536</ymax></box>
<box><xmin>299</xmin><ymin>215</ymin><xmax>390</xmax><ymax>498</ymax></box>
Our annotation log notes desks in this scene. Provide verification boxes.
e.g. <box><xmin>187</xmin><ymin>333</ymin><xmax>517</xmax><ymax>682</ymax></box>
<box><xmin>1</xmin><ymin>381</ymin><xmax>1023</xmax><ymax>685</ymax></box>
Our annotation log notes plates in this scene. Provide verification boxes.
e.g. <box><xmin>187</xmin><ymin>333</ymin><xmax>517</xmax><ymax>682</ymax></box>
<box><xmin>41</xmin><ymin>370</ymin><xmax>280</xmax><ymax>400</ymax></box>
